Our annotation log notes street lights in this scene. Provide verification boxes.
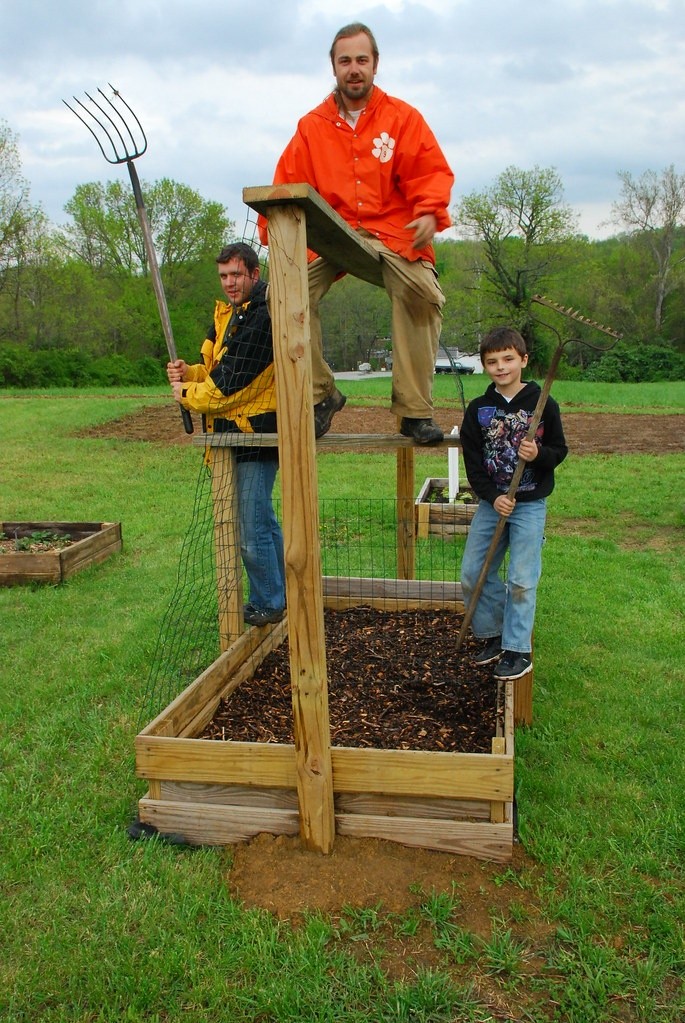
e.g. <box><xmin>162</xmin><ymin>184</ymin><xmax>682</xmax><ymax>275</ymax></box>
<box><xmin>472</xmin><ymin>257</ymin><xmax>481</xmax><ymax>353</ymax></box>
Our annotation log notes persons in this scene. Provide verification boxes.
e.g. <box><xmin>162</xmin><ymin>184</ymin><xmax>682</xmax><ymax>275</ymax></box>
<box><xmin>166</xmin><ymin>242</ymin><xmax>287</xmax><ymax>625</ymax></box>
<box><xmin>258</xmin><ymin>24</ymin><xmax>455</xmax><ymax>446</ymax></box>
<box><xmin>459</xmin><ymin>328</ymin><xmax>569</xmax><ymax>680</ymax></box>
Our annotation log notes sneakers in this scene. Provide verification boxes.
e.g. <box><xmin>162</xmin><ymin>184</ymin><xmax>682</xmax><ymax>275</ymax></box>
<box><xmin>399</xmin><ymin>417</ymin><xmax>444</xmax><ymax>445</ymax></box>
<box><xmin>242</xmin><ymin>604</ymin><xmax>284</xmax><ymax>627</ymax></box>
<box><xmin>474</xmin><ymin>637</ymin><xmax>506</xmax><ymax>665</ymax></box>
<box><xmin>314</xmin><ymin>389</ymin><xmax>347</xmax><ymax>439</ymax></box>
<box><xmin>493</xmin><ymin>650</ymin><xmax>534</xmax><ymax>681</ymax></box>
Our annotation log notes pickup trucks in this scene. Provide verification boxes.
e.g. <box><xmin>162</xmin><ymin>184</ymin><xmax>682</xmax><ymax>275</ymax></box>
<box><xmin>435</xmin><ymin>362</ymin><xmax>475</xmax><ymax>375</ymax></box>
